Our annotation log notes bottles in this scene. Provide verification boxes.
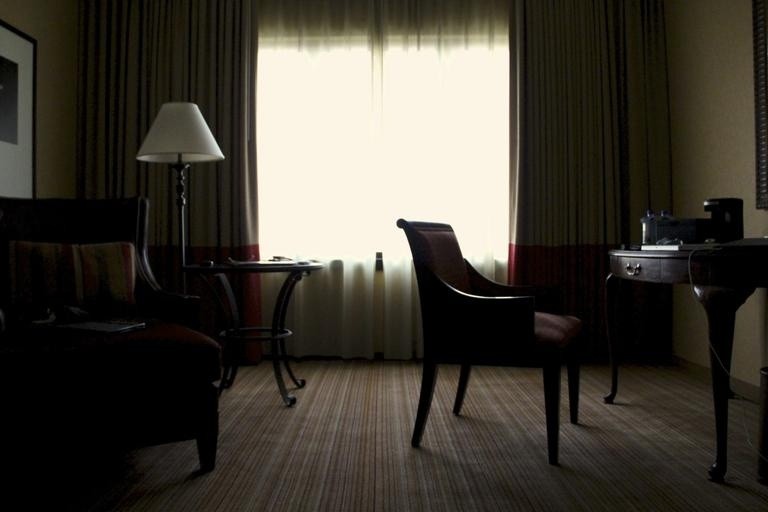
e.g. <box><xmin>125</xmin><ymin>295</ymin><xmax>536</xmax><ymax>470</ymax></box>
<box><xmin>639</xmin><ymin>209</ymin><xmax>675</xmax><ymax>244</ymax></box>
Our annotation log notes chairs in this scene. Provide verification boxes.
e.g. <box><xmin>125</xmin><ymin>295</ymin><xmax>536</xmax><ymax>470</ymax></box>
<box><xmin>0</xmin><ymin>196</ymin><xmax>221</xmax><ymax>498</ymax></box>
<box><xmin>398</xmin><ymin>219</ymin><xmax>583</xmax><ymax>465</ymax></box>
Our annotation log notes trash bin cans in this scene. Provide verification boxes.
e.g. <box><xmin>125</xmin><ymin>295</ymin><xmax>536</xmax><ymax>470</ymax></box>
<box><xmin>758</xmin><ymin>366</ymin><xmax>767</xmax><ymax>487</ymax></box>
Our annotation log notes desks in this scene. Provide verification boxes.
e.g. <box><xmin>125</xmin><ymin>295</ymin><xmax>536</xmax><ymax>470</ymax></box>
<box><xmin>181</xmin><ymin>260</ymin><xmax>324</xmax><ymax>406</ymax></box>
<box><xmin>603</xmin><ymin>239</ymin><xmax>767</xmax><ymax>484</ymax></box>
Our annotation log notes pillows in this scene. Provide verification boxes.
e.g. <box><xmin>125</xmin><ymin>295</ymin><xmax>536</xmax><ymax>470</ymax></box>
<box><xmin>0</xmin><ymin>240</ymin><xmax>136</xmax><ymax>307</ymax></box>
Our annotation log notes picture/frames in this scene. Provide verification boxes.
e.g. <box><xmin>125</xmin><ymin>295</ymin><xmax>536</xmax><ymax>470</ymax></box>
<box><xmin>0</xmin><ymin>18</ymin><xmax>38</xmax><ymax>200</ymax></box>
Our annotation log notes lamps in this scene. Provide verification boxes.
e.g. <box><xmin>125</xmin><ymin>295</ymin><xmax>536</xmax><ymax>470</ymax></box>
<box><xmin>135</xmin><ymin>101</ymin><xmax>227</xmax><ymax>295</ymax></box>
